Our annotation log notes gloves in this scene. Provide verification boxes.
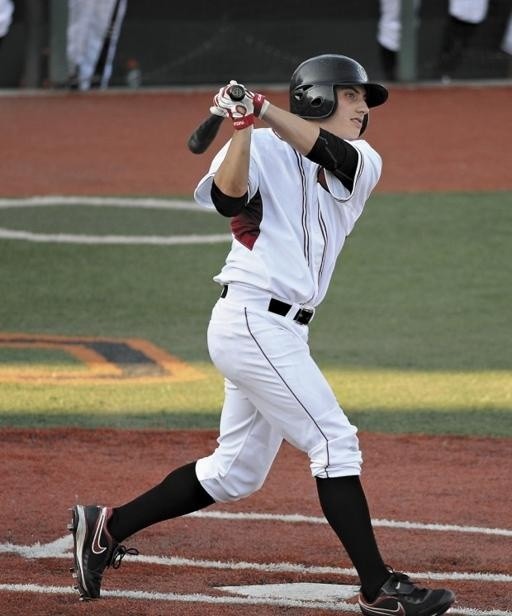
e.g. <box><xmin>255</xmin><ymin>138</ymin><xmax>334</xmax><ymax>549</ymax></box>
<box><xmin>210</xmin><ymin>80</ymin><xmax>267</xmax><ymax>130</ymax></box>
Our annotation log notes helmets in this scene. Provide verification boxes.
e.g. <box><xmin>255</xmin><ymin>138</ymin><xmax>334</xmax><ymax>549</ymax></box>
<box><xmin>289</xmin><ymin>55</ymin><xmax>389</xmax><ymax>139</ymax></box>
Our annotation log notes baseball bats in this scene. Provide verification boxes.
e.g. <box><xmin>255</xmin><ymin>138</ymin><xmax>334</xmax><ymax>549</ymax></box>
<box><xmin>188</xmin><ymin>85</ymin><xmax>245</xmax><ymax>154</ymax></box>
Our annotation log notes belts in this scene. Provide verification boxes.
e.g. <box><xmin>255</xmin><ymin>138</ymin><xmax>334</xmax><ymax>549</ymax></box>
<box><xmin>221</xmin><ymin>284</ymin><xmax>313</xmax><ymax>324</ymax></box>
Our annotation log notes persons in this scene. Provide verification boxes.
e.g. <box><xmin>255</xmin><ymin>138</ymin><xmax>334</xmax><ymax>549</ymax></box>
<box><xmin>64</xmin><ymin>51</ymin><xmax>457</xmax><ymax>616</ymax></box>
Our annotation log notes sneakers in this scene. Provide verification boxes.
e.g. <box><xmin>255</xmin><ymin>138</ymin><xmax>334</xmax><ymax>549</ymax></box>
<box><xmin>357</xmin><ymin>564</ymin><xmax>456</xmax><ymax>616</ymax></box>
<box><xmin>67</xmin><ymin>505</ymin><xmax>140</xmax><ymax>602</ymax></box>
